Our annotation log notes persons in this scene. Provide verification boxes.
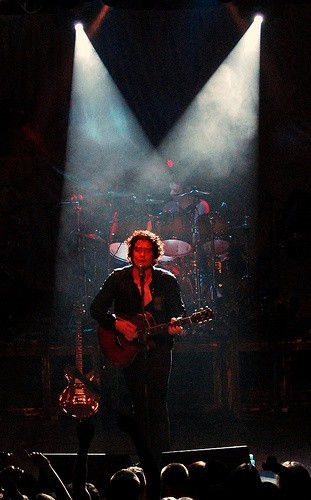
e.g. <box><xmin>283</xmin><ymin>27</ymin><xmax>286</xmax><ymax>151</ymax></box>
<box><xmin>164</xmin><ymin>170</ymin><xmax>209</xmax><ymax>222</ymax></box>
<box><xmin>73</xmin><ymin>420</ymin><xmax>189</xmax><ymax>500</ymax></box>
<box><xmin>0</xmin><ymin>449</ymin><xmax>73</xmax><ymax>500</ymax></box>
<box><xmin>90</xmin><ymin>229</ymin><xmax>189</xmax><ymax>453</ymax></box>
<box><xmin>188</xmin><ymin>461</ymin><xmax>311</xmax><ymax>500</ymax></box>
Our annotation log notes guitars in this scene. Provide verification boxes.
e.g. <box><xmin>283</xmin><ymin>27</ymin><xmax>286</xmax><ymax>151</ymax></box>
<box><xmin>58</xmin><ymin>301</ymin><xmax>103</xmax><ymax>419</ymax></box>
<box><xmin>97</xmin><ymin>305</ymin><xmax>215</xmax><ymax>368</ymax></box>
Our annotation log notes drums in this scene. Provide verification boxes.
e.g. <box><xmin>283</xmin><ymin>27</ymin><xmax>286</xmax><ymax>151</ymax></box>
<box><xmin>107</xmin><ymin>203</ymin><xmax>154</xmax><ymax>264</ymax></box>
<box><xmin>154</xmin><ymin>210</ymin><xmax>194</xmax><ymax>256</ymax></box>
<box><xmin>195</xmin><ymin>212</ymin><xmax>229</xmax><ymax>254</ymax></box>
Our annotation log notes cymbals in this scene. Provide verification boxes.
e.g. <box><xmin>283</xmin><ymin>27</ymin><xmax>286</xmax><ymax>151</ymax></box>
<box><xmin>137</xmin><ymin>198</ymin><xmax>165</xmax><ymax>204</ymax></box>
<box><xmin>178</xmin><ymin>189</ymin><xmax>212</xmax><ymax>196</ymax></box>
